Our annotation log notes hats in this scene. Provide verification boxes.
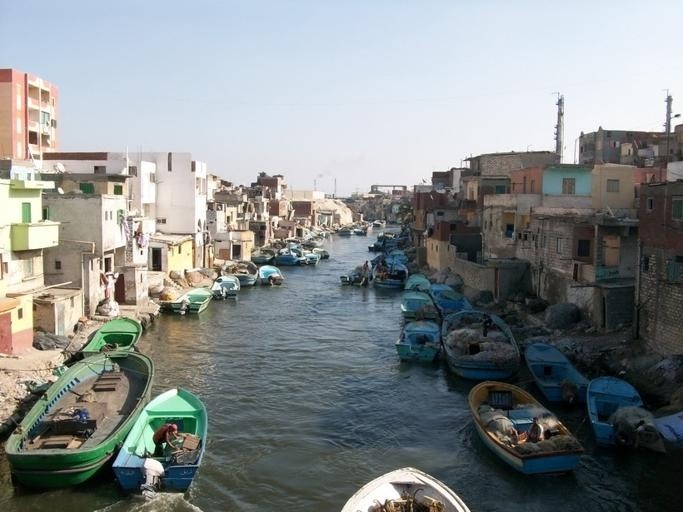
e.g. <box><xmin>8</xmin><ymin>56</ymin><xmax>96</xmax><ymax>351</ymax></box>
<box><xmin>167</xmin><ymin>423</ymin><xmax>178</xmax><ymax>435</ymax></box>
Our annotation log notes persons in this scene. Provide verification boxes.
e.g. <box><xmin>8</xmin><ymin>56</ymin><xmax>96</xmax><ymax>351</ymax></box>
<box><xmin>525</xmin><ymin>416</ymin><xmax>545</xmax><ymax>445</ymax></box>
<box><xmin>150</xmin><ymin>423</ymin><xmax>181</xmax><ymax>458</ymax></box>
